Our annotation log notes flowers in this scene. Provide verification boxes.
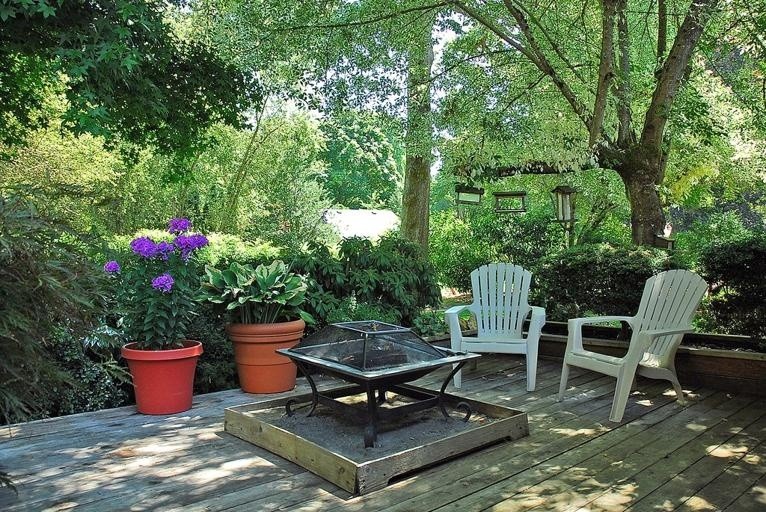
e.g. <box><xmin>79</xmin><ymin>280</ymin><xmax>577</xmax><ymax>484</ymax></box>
<box><xmin>104</xmin><ymin>217</ymin><xmax>208</xmax><ymax>351</ymax></box>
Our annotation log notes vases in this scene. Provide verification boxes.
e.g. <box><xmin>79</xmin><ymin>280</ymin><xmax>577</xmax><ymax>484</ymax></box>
<box><xmin>121</xmin><ymin>340</ymin><xmax>202</xmax><ymax>415</ymax></box>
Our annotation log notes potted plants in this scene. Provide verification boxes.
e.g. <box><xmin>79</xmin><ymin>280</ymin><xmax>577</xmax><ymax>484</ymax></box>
<box><xmin>204</xmin><ymin>257</ymin><xmax>327</xmax><ymax>394</ymax></box>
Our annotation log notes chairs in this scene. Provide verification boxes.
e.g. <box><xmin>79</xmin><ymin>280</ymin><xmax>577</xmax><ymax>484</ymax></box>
<box><xmin>442</xmin><ymin>261</ymin><xmax>546</xmax><ymax>391</ymax></box>
<box><xmin>559</xmin><ymin>269</ymin><xmax>711</xmax><ymax>423</ymax></box>
